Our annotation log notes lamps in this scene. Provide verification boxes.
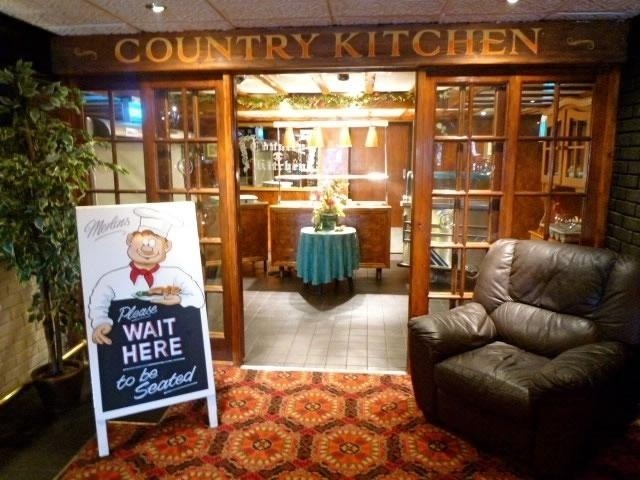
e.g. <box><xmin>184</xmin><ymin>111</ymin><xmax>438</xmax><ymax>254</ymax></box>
<box><xmin>272</xmin><ymin>119</ymin><xmax>388</xmax><ymax>147</ymax></box>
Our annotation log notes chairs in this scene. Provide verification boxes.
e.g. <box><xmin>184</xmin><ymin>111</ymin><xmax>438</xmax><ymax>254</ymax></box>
<box><xmin>406</xmin><ymin>237</ymin><xmax>640</xmax><ymax>480</ymax></box>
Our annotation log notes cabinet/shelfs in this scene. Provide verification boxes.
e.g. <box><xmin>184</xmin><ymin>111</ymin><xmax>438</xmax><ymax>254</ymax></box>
<box><xmin>528</xmin><ymin>230</ymin><xmax>545</xmax><ymax>240</ymax></box>
<box><xmin>539</xmin><ymin>96</ymin><xmax>592</xmax><ymax>193</ymax></box>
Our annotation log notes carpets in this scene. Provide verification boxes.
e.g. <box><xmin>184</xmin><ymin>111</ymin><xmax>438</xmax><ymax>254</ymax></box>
<box><xmin>55</xmin><ymin>361</ymin><xmax>535</xmax><ymax>480</ymax></box>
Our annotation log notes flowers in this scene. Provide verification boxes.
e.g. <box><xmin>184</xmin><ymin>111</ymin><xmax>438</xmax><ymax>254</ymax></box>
<box><xmin>310</xmin><ymin>177</ymin><xmax>352</xmax><ymax>231</ymax></box>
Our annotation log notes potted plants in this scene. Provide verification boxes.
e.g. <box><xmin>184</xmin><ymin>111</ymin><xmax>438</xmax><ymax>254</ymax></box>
<box><xmin>0</xmin><ymin>57</ymin><xmax>129</xmax><ymax>415</ymax></box>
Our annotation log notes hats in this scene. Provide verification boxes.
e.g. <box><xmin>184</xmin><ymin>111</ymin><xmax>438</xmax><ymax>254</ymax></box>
<box><xmin>134</xmin><ymin>207</ymin><xmax>182</xmax><ymax>238</ymax></box>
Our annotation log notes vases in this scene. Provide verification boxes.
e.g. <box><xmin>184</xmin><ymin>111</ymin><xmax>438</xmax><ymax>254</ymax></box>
<box><xmin>320</xmin><ymin>212</ymin><xmax>338</xmax><ymax>231</ymax></box>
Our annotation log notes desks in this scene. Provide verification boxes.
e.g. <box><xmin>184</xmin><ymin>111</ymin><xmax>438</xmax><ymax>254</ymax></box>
<box><xmin>269</xmin><ymin>201</ymin><xmax>391</xmax><ymax>282</ymax></box>
<box><xmin>294</xmin><ymin>226</ymin><xmax>361</xmax><ymax>299</ymax></box>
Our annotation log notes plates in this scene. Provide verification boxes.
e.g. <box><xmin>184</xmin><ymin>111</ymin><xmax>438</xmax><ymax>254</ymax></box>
<box><xmin>131</xmin><ymin>289</ymin><xmax>165</xmax><ymax>302</ymax></box>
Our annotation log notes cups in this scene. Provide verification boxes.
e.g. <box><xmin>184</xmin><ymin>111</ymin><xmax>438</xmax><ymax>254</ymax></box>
<box><xmin>473</xmin><ymin>154</ymin><xmax>496</xmax><ymax>176</ymax></box>
<box><xmin>197</xmin><ymin>208</ymin><xmax>213</xmax><ymax>227</ymax></box>
<box><xmin>439</xmin><ymin>214</ymin><xmax>454</xmax><ymax>231</ymax></box>
<box><xmin>168</xmin><ymin>111</ymin><xmax>180</xmax><ymax>127</ymax></box>
